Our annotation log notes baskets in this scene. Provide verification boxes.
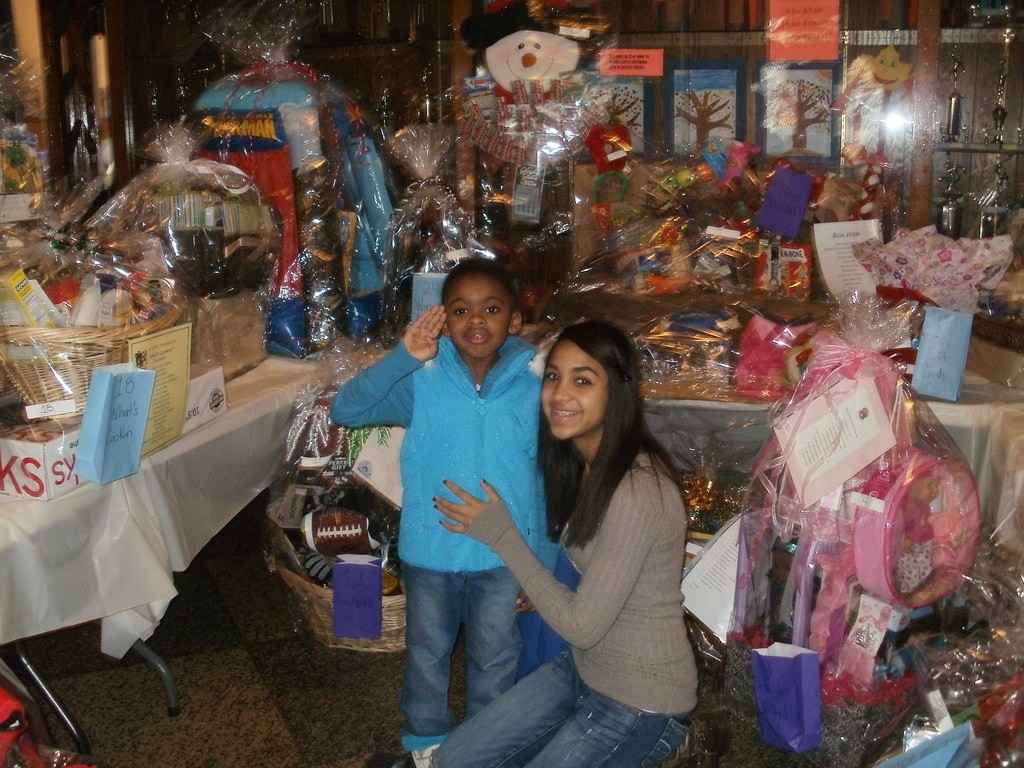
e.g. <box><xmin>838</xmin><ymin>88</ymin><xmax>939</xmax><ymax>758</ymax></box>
<box><xmin>0</xmin><ymin>273</ymin><xmax>189</xmax><ymax>418</ymax></box>
<box><xmin>266</xmin><ymin>553</ymin><xmax>409</xmax><ymax>654</ymax></box>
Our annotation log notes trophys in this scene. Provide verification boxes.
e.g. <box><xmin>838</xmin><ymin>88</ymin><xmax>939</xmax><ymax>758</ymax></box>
<box><xmin>967</xmin><ymin>0</ymin><xmax>1016</xmax><ymax>28</ymax></box>
<box><xmin>946</xmin><ymin>43</ymin><xmax>966</xmax><ymax>143</ymax></box>
<box><xmin>321</xmin><ymin>0</ymin><xmax>433</xmax><ymax>133</ymax></box>
<box><xmin>937</xmin><ymin>159</ymin><xmax>967</xmax><ymax>241</ymax></box>
<box><xmin>977</xmin><ymin>163</ymin><xmax>1010</xmax><ymax>240</ymax></box>
<box><xmin>992</xmin><ymin>58</ymin><xmax>1008</xmax><ymax>143</ymax></box>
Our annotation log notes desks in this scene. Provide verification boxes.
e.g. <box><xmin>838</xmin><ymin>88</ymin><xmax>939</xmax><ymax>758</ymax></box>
<box><xmin>334</xmin><ymin>339</ymin><xmax>1024</xmax><ymax>554</ymax></box>
<box><xmin>0</xmin><ymin>356</ymin><xmax>333</xmax><ymax>721</ymax></box>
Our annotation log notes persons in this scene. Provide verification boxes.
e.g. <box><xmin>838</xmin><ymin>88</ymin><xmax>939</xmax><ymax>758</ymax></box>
<box><xmin>766</xmin><ymin>543</ymin><xmax>797</xmax><ymax>646</ymax></box>
<box><xmin>329</xmin><ymin>258</ymin><xmax>562</xmax><ymax>768</ymax></box>
<box><xmin>430</xmin><ymin>319</ymin><xmax>699</xmax><ymax>768</ymax></box>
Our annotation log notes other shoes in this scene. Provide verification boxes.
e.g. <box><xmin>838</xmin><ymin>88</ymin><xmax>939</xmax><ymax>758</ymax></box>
<box><xmin>411</xmin><ymin>744</ymin><xmax>443</xmax><ymax>768</ymax></box>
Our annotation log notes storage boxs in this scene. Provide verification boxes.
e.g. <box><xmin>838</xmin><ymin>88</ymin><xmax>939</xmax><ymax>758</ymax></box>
<box><xmin>0</xmin><ymin>267</ymin><xmax>55</xmax><ymax>328</ymax></box>
<box><xmin>837</xmin><ymin>583</ymin><xmax>913</xmax><ymax>685</ymax></box>
<box><xmin>0</xmin><ymin>192</ymin><xmax>44</xmax><ymax>223</ymax></box>
<box><xmin>0</xmin><ymin>363</ymin><xmax>229</xmax><ymax>502</ymax></box>
<box><xmin>150</xmin><ymin>193</ymin><xmax>275</xmax><ymax>261</ymax></box>
<box><xmin>99</xmin><ymin>289</ymin><xmax>133</xmax><ymax>326</ymax></box>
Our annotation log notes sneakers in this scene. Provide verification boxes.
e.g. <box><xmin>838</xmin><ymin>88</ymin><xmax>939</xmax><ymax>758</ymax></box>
<box><xmin>659</xmin><ymin>711</ymin><xmax>731</xmax><ymax>768</ymax></box>
